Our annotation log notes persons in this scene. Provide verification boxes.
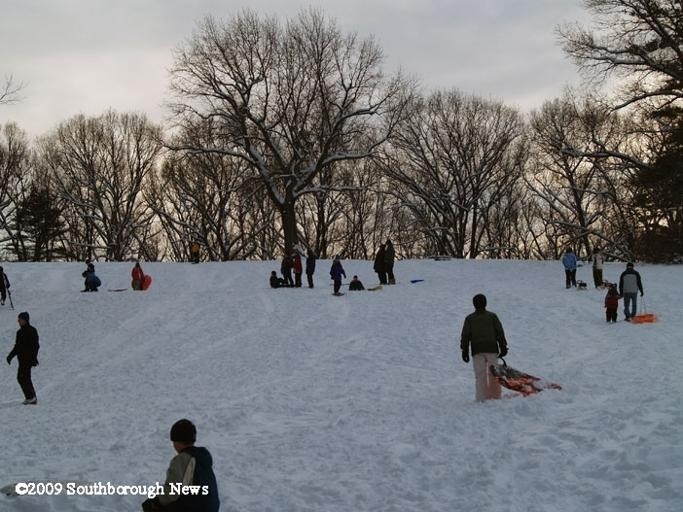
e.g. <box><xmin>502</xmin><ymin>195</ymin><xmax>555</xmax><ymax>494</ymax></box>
<box><xmin>0</xmin><ymin>267</ymin><xmax>10</xmax><ymax>305</ymax></box>
<box><xmin>80</xmin><ymin>271</ymin><xmax>101</xmax><ymax>291</ymax></box>
<box><xmin>131</xmin><ymin>263</ymin><xmax>145</xmax><ymax>290</ymax></box>
<box><xmin>141</xmin><ymin>417</ymin><xmax>218</xmax><ymax>510</ymax></box>
<box><xmin>269</xmin><ymin>239</ymin><xmax>395</xmax><ymax>296</ymax></box>
<box><xmin>561</xmin><ymin>246</ymin><xmax>577</xmax><ymax>289</ymax></box>
<box><xmin>190</xmin><ymin>241</ymin><xmax>200</xmax><ymax>264</ymax></box>
<box><xmin>619</xmin><ymin>262</ymin><xmax>643</xmax><ymax>321</ymax></box>
<box><xmin>603</xmin><ymin>284</ymin><xmax>619</xmax><ymax>323</ymax></box>
<box><xmin>6</xmin><ymin>312</ymin><xmax>39</xmax><ymax>404</ymax></box>
<box><xmin>460</xmin><ymin>293</ymin><xmax>508</xmax><ymax>402</ymax></box>
<box><xmin>591</xmin><ymin>248</ymin><xmax>604</xmax><ymax>288</ymax></box>
<box><xmin>85</xmin><ymin>260</ymin><xmax>94</xmax><ymax>273</ymax></box>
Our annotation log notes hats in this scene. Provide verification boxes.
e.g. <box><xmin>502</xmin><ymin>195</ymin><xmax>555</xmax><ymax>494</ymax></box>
<box><xmin>473</xmin><ymin>293</ymin><xmax>487</xmax><ymax>309</ymax></box>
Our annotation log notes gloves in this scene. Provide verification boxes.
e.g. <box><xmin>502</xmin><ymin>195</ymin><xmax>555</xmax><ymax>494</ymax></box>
<box><xmin>462</xmin><ymin>350</ymin><xmax>470</xmax><ymax>363</ymax></box>
<box><xmin>498</xmin><ymin>348</ymin><xmax>509</xmax><ymax>357</ymax></box>
